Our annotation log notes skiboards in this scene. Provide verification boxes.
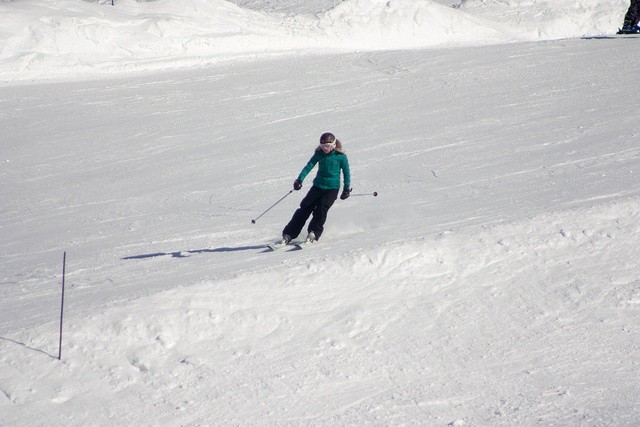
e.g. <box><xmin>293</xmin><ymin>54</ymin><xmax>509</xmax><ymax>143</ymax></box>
<box><xmin>267</xmin><ymin>238</ymin><xmax>310</xmax><ymax>251</ymax></box>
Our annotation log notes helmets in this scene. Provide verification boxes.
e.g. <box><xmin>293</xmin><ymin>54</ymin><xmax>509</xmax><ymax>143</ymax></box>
<box><xmin>320</xmin><ymin>133</ymin><xmax>336</xmax><ymax>147</ymax></box>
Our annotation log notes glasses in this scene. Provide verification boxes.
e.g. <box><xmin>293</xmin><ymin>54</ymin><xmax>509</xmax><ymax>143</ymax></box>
<box><xmin>320</xmin><ymin>138</ymin><xmax>336</xmax><ymax>149</ymax></box>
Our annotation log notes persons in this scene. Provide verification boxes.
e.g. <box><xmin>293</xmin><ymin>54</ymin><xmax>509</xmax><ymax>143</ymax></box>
<box><xmin>273</xmin><ymin>133</ymin><xmax>353</xmax><ymax>248</ymax></box>
<box><xmin>621</xmin><ymin>0</ymin><xmax>640</xmax><ymax>30</ymax></box>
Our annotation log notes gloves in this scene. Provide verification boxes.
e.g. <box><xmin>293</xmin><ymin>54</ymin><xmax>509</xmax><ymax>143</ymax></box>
<box><xmin>294</xmin><ymin>180</ymin><xmax>302</xmax><ymax>190</ymax></box>
<box><xmin>340</xmin><ymin>188</ymin><xmax>352</xmax><ymax>199</ymax></box>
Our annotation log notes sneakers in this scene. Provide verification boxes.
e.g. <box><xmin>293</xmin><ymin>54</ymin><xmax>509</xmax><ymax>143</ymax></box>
<box><xmin>305</xmin><ymin>232</ymin><xmax>315</xmax><ymax>244</ymax></box>
<box><xmin>280</xmin><ymin>234</ymin><xmax>291</xmax><ymax>245</ymax></box>
